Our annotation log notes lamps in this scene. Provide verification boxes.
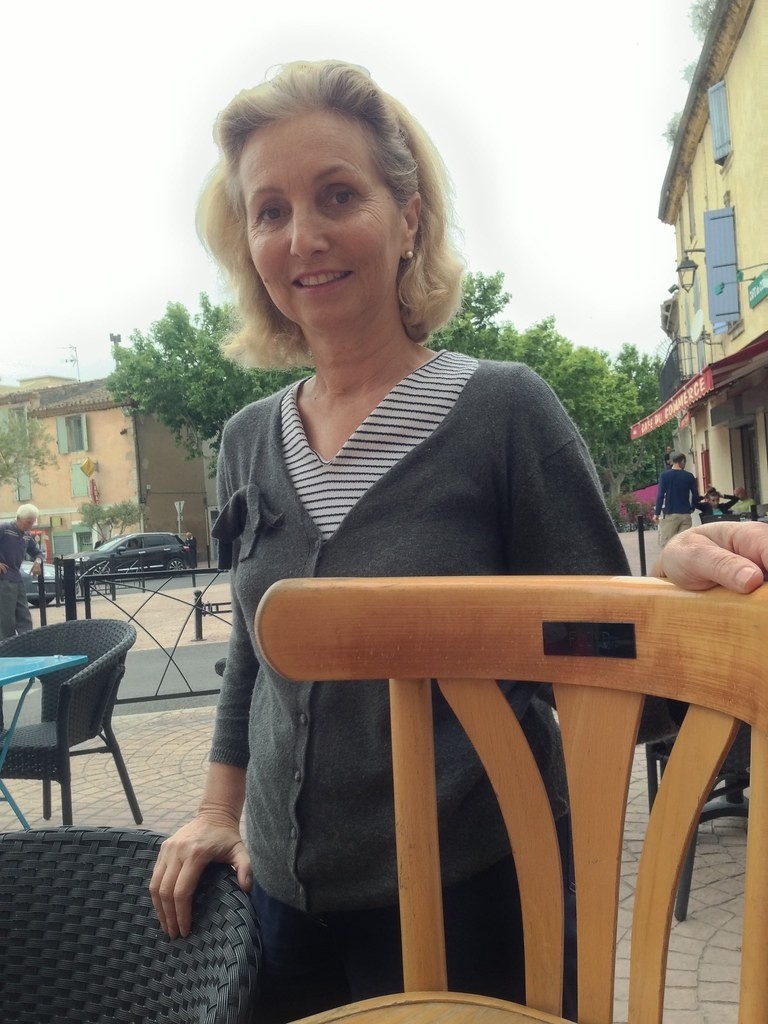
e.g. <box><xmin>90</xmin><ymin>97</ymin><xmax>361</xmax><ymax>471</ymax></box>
<box><xmin>676</xmin><ymin>249</ymin><xmax>708</xmax><ymax>294</ymax></box>
<box><xmin>667</xmin><ymin>283</ymin><xmax>679</xmax><ymax>295</ymax></box>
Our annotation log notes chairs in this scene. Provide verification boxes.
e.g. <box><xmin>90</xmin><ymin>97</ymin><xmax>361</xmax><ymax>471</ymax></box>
<box><xmin>701</xmin><ymin>514</ymin><xmax>741</xmax><ymax>524</ymax></box>
<box><xmin>646</xmin><ymin>727</ymin><xmax>751</xmax><ymax>923</ymax></box>
<box><xmin>0</xmin><ymin>617</ymin><xmax>144</xmax><ymax>828</ymax></box>
<box><xmin>253</xmin><ymin>571</ymin><xmax>768</xmax><ymax>1024</ymax></box>
<box><xmin>0</xmin><ymin>823</ymin><xmax>259</xmax><ymax>1024</ymax></box>
<box><xmin>751</xmin><ymin>503</ymin><xmax>768</xmax><ymax>521</ymax></box>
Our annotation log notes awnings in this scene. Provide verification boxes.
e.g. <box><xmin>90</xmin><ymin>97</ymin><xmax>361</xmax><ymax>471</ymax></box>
<box><xmin>629</xmin><ymin>338</ymin><xmax>768</xmax><ymax>440</ymax></box>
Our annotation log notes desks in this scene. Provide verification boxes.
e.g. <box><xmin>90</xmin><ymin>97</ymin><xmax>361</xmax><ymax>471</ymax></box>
<box><xmin>0</xmin><ymin>653</ymin><xmax>90</xmax><ymax>832</ymax></box>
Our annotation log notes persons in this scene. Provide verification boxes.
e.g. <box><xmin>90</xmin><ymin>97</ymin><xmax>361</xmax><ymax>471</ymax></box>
<box><xmin>653</xmin><ymin>445</ymin><xmax>757</xmax><ymax>548</ymax></box>
<box><xmin>146</xmin><ymin>59</ymin><xmax>768</xmax><ymax>1024</ymax></box>
<box><xmin>128</xmin><ymin>538</ymin><xmax>138</xmax><ymax>549</ymax></box>
<box><xmin>184</xmin><ymin>531</ymin><xmax>196</xmax><ymax>569</ymax></box>
<box><xmin>0</xmin><ymin>503</ymin><xmax>45</xmax><ymax>641</ymax></box>
<box><xmin>95</xmin><ymin>536</ymin><xmax>105</xmax><ymax>550</ymax></box>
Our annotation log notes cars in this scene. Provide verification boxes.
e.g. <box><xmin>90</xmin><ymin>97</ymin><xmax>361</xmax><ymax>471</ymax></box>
<box><xmin>52</xmin><ymin>533</ymin><xmax>191</xmax><ymax>585</ymax></box>
<box><xmin>19</xmin><ymin>560</ymin><xmax>80</xmax><ymax>606</ymax></box>
